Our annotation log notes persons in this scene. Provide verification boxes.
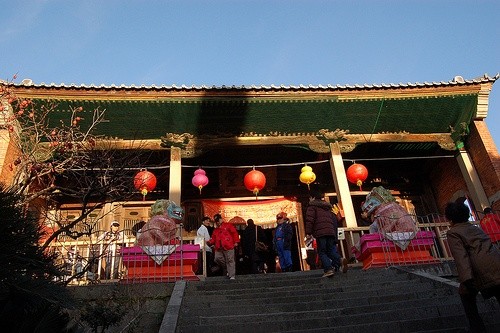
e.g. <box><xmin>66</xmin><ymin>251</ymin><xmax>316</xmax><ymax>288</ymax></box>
<box><xmin>197</xmin><ymin>214</ymin><xmax>278</xmax><ymax>280</ymax></box>
<box><xmin>444</xmin><ymin>196</ymin><xmax>500</xmax><ymax>333</ymax></box>
<box><xmin>97</xmin><ymin>221</ymin><xmax>130</xmax><ymax>279</ymax></box>
<box><xmin>480</xmin><ymin>207</ymin><xmax>500</xmax><ymax>249</ymax></box>
<box><xmin>305</xmin><ymin>190</ymin><xmax>347</xmax><ymax>278</ymax></box>
<box><xmin>273</xmin><ymin>213</ymin><xmax>294</xmax><ymax>272</ymax></box>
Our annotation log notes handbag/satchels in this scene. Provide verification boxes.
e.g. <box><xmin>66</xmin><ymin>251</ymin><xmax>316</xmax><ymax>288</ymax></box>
<box><xmin>254</xmin><ymin>241</ymin><xmax>269</xmax><ymax>257</ymax></box>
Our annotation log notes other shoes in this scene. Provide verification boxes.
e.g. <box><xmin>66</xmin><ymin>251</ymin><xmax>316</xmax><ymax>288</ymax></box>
<box><xmin>322</xmin><ymin>270</ymin><xmax>334</xmax><ymax>278</ymax></box>
<box><xmin>340</xmin><ymin>257</ymin><xmax>348</xmax><ymax>274</ymax></box>
<box><xmin>226</xmin><ymin>272</ymin><xmax>235</xmax><ymax>280</ymax></box>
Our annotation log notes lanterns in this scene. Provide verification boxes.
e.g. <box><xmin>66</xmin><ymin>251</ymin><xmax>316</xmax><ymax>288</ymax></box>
<box><xmin>134</xmin><ymin>169</ymin><xmax>157</xmax><ymax>196</ymax></box>
<box><xmin>300</xmin><ymin>166</ymin><xmax>316</xmax><ymax>189</ymax></box>
<box><xmin>192</xmin><ymin>168</ymin><xmax>209</xmax><ymax>193</ymax></box>
<box><xmin>347</xmin><ymin>163</ymin><xmax>369</xmax><ymax>186</ymax></box>
<box><xmin>244</xmin><ymin>170</ymin><xmax>266</xmax><ymax>197</ymax></box>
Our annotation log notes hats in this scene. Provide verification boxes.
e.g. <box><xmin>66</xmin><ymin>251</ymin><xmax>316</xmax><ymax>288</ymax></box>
<box><xmin>214</xmin><ymin>214</ymin><xmax>221</xmax><ymax>221</ymax></box>
<box><xmin>276</xmin><ymin>214</ymin><xmax>283</xmax><ymax>219</ymax></box>
